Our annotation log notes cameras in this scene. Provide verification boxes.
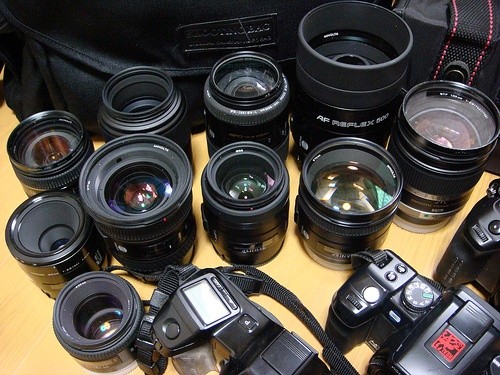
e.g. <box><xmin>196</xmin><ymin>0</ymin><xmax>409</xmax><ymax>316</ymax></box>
<box><xmin>148</xmin><ymin>267</ymin><xmax>319</xmax><ymax>375</ymax></box>
<box><xmin>321</xmin><ymin>248</ymin><xmax>500</xmax><ymax>375</ymax></box>
<box><xmin>432</xmin><ymin>177</ymin><xmax>500</xmax><ymax>312</ymax></box>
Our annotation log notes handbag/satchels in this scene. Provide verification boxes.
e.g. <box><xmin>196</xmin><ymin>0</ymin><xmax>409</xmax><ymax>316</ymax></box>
<box><xmin>0</xmin><ymin>0</ymin><xmax>314</xmax><ymax>134</ymax></box>
<box><xmin>392</xmin><ymin>0</ymin><xmax>500</xmax><ymax>174</ymax></box>
